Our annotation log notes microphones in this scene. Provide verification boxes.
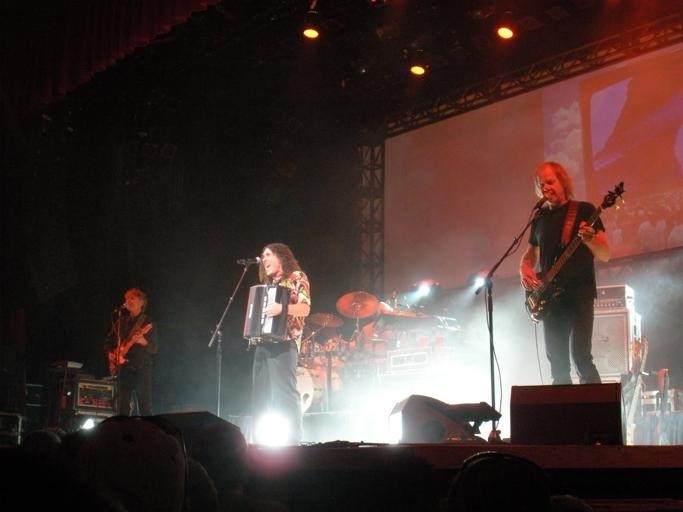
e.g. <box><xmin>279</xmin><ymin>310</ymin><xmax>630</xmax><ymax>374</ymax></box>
<box><xmin>237</xmin><ymin>256</ymin><xmax>261</xmax><ymax>265</ymax></box>
<box><xmin>532</xmin><ymin>190</ymin><xmax>551</xmax><ymax>210</ymax></box>
<box><xmin>114</xmin><ymin>302</ymin><xmax>128</xmax><ymax>312</ymax></box>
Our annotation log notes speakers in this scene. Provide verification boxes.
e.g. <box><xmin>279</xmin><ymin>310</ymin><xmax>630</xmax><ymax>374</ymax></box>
<box><xmin>510</xmin><ymin>383</ymin><xmax>627</xmax><ymax>445</ymax></box>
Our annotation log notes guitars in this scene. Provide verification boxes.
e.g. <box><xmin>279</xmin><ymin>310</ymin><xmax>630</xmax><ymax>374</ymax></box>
<box><xmin>108</xmin><ymin>323</ymin><xmax>154</xmax><ymax>376</ymax></box>
<box><xmin>525</xmin><ymin>178</ymin><xmax>625</xmax><ymax>321</ymax></box>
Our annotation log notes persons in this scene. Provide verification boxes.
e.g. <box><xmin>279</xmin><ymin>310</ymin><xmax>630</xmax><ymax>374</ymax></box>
<box><xmin>250</xmin><ymin>242</ymin><xmax>311</xmax><ymax>445</ymax></box>
<box><xmin>519</xmin><ymin>162</ymin><xmax>611</xmax><ymax>386</ymax></box>
<box><xmin>103</xmin><ymin>287</ymin><xmax>158</xmax><ymax>416</ymax></box>
<box><xmin>1</xmin><ymin>415</ymin><xmax>593</xmax><ymax>512</ymax></box>
<box><xmin>332</xmin><ymin>313</ymin><xmax>399</xmax><ymax>386</ymax></box>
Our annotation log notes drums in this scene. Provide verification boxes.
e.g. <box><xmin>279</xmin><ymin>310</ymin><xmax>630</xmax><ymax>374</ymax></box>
<box><xmin>359</xmin><ymin>321</ymin><xmax>394</xmax><ymax>376</ymax></box>
<box><xmin>296</xmin><ymin>366</ymin><xmax>343</xmax><ymax>413</ymax></box>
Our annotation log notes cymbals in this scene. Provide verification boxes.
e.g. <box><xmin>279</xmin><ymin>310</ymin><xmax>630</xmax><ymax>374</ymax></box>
<box><xmin>311</xmin><ymin>312</ymin><xmax>343</xmax><ymax>328</ymax></box>
<box><xmin>336</xmin><ymin>291</ymin><xmax>379</xmax><ymax>319</ymax></box>
